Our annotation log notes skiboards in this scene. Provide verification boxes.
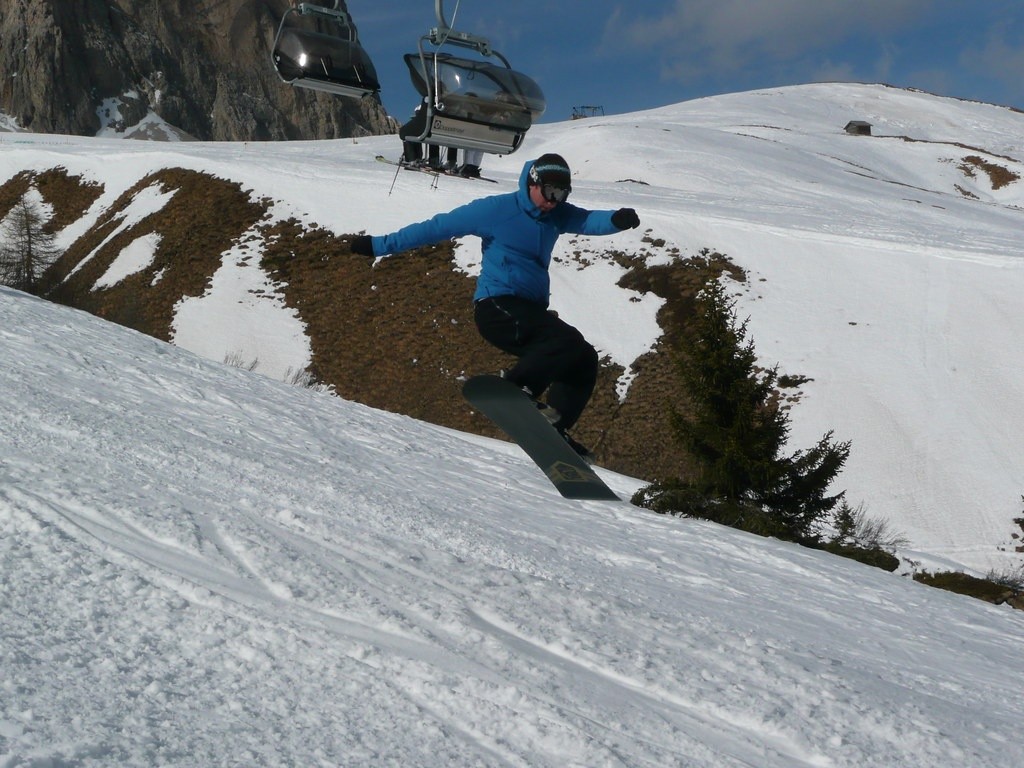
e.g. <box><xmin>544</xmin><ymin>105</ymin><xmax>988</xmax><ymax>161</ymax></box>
<box><xmin>375</xmin><ymin>153</ymin><xmax>498</xmax><ymax>185</ymax></box>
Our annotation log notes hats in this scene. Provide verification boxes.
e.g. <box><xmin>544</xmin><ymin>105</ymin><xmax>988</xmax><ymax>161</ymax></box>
<box><xmin>527</xmin><ymin>154</ymin><xmax>571</xmax><ymax>190</ymax></box>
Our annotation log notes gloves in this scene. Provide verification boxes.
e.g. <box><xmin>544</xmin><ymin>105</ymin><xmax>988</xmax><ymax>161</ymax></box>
<box><xmin>612</xmin><ymin>208</ymin><xmax>640</xmax><ymax>230</ymax></box>
<box><xmin>350</xmin><ymin>235</ymin><xmax>374</xmax><ymax>257</ymax></box>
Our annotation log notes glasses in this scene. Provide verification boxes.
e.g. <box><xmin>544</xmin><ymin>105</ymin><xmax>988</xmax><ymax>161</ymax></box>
<box><xmin>541</xmin><ymin>184</ymin><xmax>568</xmax><ymax>203</ymax></box>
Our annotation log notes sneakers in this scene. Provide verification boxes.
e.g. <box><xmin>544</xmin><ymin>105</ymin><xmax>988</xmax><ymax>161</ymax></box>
<box><xmin>500</xmin><ymin>368</ymin><xmax>547</xmax><ymax>410</ymax></box>
<box><xmin>553</xmin><ymin>422</ymin><xmax>589</xmax><ymax>457</ymax></box>
<box><xmin>402</xmin><ymin>158</ymin><xmax>482</xmax><ymax>177</ymax></box>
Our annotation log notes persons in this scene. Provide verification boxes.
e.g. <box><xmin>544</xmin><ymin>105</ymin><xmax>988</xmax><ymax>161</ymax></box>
<box><xmin>344</xmin><ymin>153</ymin><xmax>642</xmax><ymax>463</ymax></box>
<box><xmin>398</xmin><ymin>82</ymin><xmax>484</xmax><ymax>177</ymax></box>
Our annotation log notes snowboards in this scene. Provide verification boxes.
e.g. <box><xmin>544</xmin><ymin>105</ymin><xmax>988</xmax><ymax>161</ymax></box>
<box><xmin>460</xmin><ymin>373</ymin><xmax>620</xmax><ymax>501</ymax></box>
